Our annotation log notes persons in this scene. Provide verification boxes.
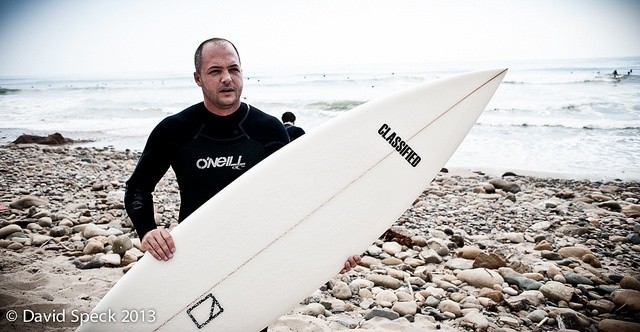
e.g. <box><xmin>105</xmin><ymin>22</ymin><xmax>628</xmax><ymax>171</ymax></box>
<box><xmin>282</xmin><ymin>111</ymin><xmax>305</xmax><ymax>142</ymax></box>
<box><xmin>124</xmin><ymin>37</ymin><xmax>361</xmax><ymax>274</ymax></box>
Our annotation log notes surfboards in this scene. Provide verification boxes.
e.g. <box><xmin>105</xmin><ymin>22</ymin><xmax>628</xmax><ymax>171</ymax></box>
<box><xmin>75</xmin><ymin>67</ymin><xmax>509</xmax><ymax>332</ymax></box>
